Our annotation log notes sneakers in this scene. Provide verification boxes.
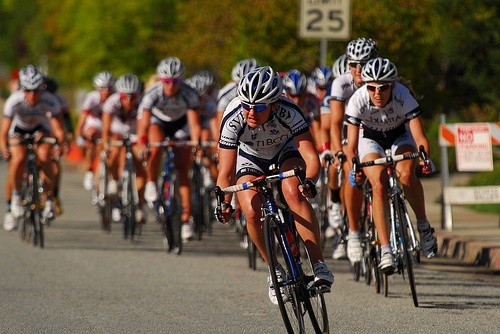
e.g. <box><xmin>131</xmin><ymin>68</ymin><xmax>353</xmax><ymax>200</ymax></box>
<box><xmin>3</xmin><ymin>193</ymin><xmax>54</xmax><ymax>231</ymax></box>
<box><xmin>83</xmin><ymin>171</ymin><xmax>218</xmax><ymax>240</ymax></box>
<box><xmin>267</xmin><ymin>196</ymin><xmax>438</xmax><ymax>305</ymax></box>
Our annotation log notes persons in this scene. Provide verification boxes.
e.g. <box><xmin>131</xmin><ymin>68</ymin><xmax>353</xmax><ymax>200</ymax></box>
<box><xmin>213</xmin><ymin>66</ymin><xmax>334</xmax><ymax>306</ymax></box>
<box><xmin>0</xmin><ymin>38</ymin><xmax>418</xmax><ymax>263</ymax></box>
<box><xmin>347</xmin><ymin>57</ymin><xmax>438</xmax><ymax>272</ymax></box>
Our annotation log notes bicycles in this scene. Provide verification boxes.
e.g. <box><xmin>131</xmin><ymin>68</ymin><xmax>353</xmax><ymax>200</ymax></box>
<box><xmin>5</xmin><ymin>130</ymin><xmax>384</xmax><ymax>294</ymax></box>
<box><xmin>351</xmin><ymin>144</ymin><xmax>428</xmax><ymax>307</ymax></box>
<box><xmin>214</xmin><ymin>163</ymin><xmax>330</xmax><ymax>334</ymax></box>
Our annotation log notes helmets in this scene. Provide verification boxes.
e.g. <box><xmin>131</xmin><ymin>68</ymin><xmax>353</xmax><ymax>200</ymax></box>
<box><xmin>156</xmin><ymin>56</ymin><xmax>184</xmax><ymax>79</ymax></box>
<box><xmin>282</xmin><ymin>69</ymin><xmax>307</xmax><ymax>95</ymax></box>
<box><xmin>311</xmin><ymin>66</ymin><xmax>333</xmax><ymax>86</ymax></box>
<box><xmin>92</xmin><ymin>71</ymin><xmax>114</xmax><ymax>88</ymax></box>
<box><xmin>346</xmin><ymin>37</ymin><xmax>377</xmax><ymax>61</ymax></box>
<box><xmin>332</xmin><ymin>54</ymin><xmax>350</xmax><ymax>78</ymax></box>
<box><xmin>184</xmin><ymin>74</ymin><xmax>207</xmax><ymax>97</ymax></box>
<box><xmin>197</xmin><ymin>69</ymin><xmax>215</xmax><ymax>86</ymax></box>
<box><xmin>231</xmin><ymin>58</ymin><xmax>259</xmax><ymax>83</ymax></box>
<box><xmin>237</xmin><ymin>65</ymin><xmax>283</xmax><ymax>104</ymax></box>
<box><xmin>361</xmin><ymin>58</ymin><xmax>398</xmax><ymax>82</ymax></box>
<box><xmin>19</xmin><ymin>64</ymin><xmax>43</xmax><ymax>90</ymax></box>
<box><xmin>115</xmin><ymin>74</ymin><xmax>141</xmax><ymax>94</ymax></box>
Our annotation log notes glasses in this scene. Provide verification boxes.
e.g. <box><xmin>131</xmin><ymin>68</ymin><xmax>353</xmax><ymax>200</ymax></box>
<box><xmin>317</xmin><ymin>85</ymin><xmax>328</xmax><ymax>90</ymax></box>
<box><xmin>348</xmin><ymin>62</ymin><xmax>366</xmax><ymax>68</ymax></box>
<box><xmin>162</xmin><ymin>80</ymin><xmax>179</xmax><ymax>84</ymax></box>
<box><xmin>367</xmin><ymin>82</ymin><xmax>390</xmax><ymax>92</ymax></box>
<box><xmin>241</xmin><ymin>102</ymin><xmax>266</xmax><ymax>113</ymax></box>
<box><xmin>288</xmin><ymin>91</ymin><xmax>304</xmax><ymax>98</ymax></box>
<box><xmin>23</xmin><ymin>89</ymin><xmax>41</xmax><ymax>93</ymax></box>
